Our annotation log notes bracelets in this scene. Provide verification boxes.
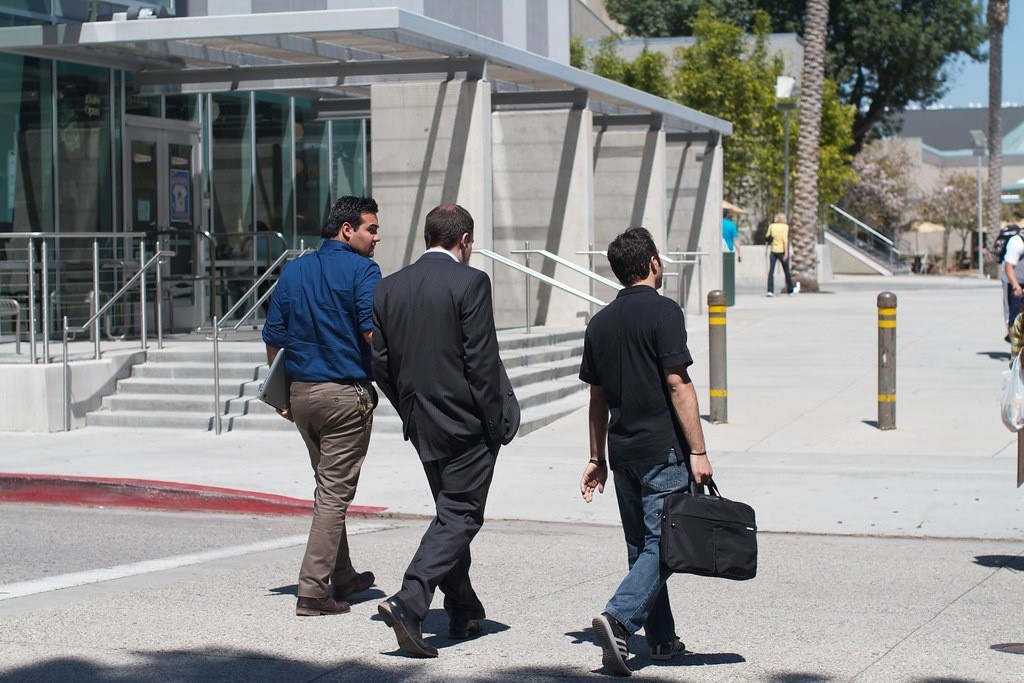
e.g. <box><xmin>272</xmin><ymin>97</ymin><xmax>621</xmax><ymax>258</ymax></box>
<box><xmin>589</xmin><ymin>459</ymin><xmax>606</xmax><ymax>466</ymax></box>
<box><xmin>690</xmin><ymin>451</ymin><xmax>706</xmax><ymax>455</ymax></box>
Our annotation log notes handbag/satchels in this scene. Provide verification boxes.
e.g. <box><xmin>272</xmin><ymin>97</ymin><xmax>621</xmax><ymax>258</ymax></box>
<box><xmin>660</xmin><ymin>473</ymin><xmax>758</xmax><ymax>580</ymax></box>
<box><xmin>999</xmin><ymin>347</ymin><xmax>1024</xmax><ymax>432</ymax></box>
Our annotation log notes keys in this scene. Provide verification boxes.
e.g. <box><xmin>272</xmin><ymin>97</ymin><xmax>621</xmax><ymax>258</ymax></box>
<box><xmin>357</xmin><ymin>395</ymin><xmax>367</xmax><ymax>416</ymax></box>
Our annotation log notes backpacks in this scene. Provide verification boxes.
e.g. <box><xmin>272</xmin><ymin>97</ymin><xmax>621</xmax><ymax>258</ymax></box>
<box><xmin>991</xmin><ymin>225</ymin><xmax>1024</xmax><ymax>264</ymax></box>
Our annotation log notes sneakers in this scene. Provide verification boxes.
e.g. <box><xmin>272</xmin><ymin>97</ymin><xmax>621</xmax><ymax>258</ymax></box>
<box><xmin>650</xmin><ymin>633</ymin><xmax>685</xmax><ymax>660</ymax></box>
<box><xmin>592</xmin><ymin>612</ymin><xmax>633</xmax><ymax>675</ymax></box>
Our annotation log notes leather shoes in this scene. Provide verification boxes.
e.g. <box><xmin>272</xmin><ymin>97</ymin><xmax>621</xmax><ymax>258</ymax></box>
<box><xmin>447</xmin><ymin>616</ymin><xmax>483</xmax><ymax>638</ymax></box>
<box><xmin>378</xmin><ymin>595</ymin><xmax>438</xmax><ymax>656</ymax></box>
<box><xmin>296</xmin><ymin>595</ymin><xmax>350</xmax><ymax>615</ymax></box>
<box><xmin>333</xmin><ymin>571</ymin><xmax>375</xmax><ymax>601</ymax></box>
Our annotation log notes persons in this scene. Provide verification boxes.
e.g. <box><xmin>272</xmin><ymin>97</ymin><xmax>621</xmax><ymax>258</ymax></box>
<box><xmin>262</xmin><ymin>195</ymin><xmax>382</xmax><ymax>617</ymax></box>
<box><xmin>580</xmin><ymin>228</ymin><xmax>713</xmax><ymax>677</ymax></box>
<box><xmin>1001</xmin><ymin>227</ymin><xmax>1024</xmax><ymax>343</ymax></box>
<box><xmin>721</xmin><ymin>208</ymin><xmax>743</xmax><ymax>263</ymax></box>
<box><xmin>765</xmin><ymin>213</ymin><xmax>797</xmax><ymax>297</ymax></box>
<box><xmin>370</xmin><ymin>204</ymin><xmax>521</xmax><ymax>657</ymax></box>
<box><xmin>1009</xmin><ymin>296</ymin><xmax>1024</xmax><ymax>371</ymax></box>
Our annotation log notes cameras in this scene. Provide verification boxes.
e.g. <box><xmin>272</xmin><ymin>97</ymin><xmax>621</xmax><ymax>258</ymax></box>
<box><xmin>765</xmin><ymin>235</ymin><xmax>774</xmax><ymax>241</ymax></box>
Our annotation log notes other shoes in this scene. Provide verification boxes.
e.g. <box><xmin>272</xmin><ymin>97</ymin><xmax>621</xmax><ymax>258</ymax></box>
<box><xmin>767</xmin><ymin>292</ymin><xmax>773</xmax><ymax>296</ymax></box>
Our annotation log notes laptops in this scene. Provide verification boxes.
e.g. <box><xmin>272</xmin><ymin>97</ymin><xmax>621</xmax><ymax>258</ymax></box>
<box><xmin>256</xmin><ymin>348</ymin><xmax>289</xmax><ymax>412</ymax></box>
